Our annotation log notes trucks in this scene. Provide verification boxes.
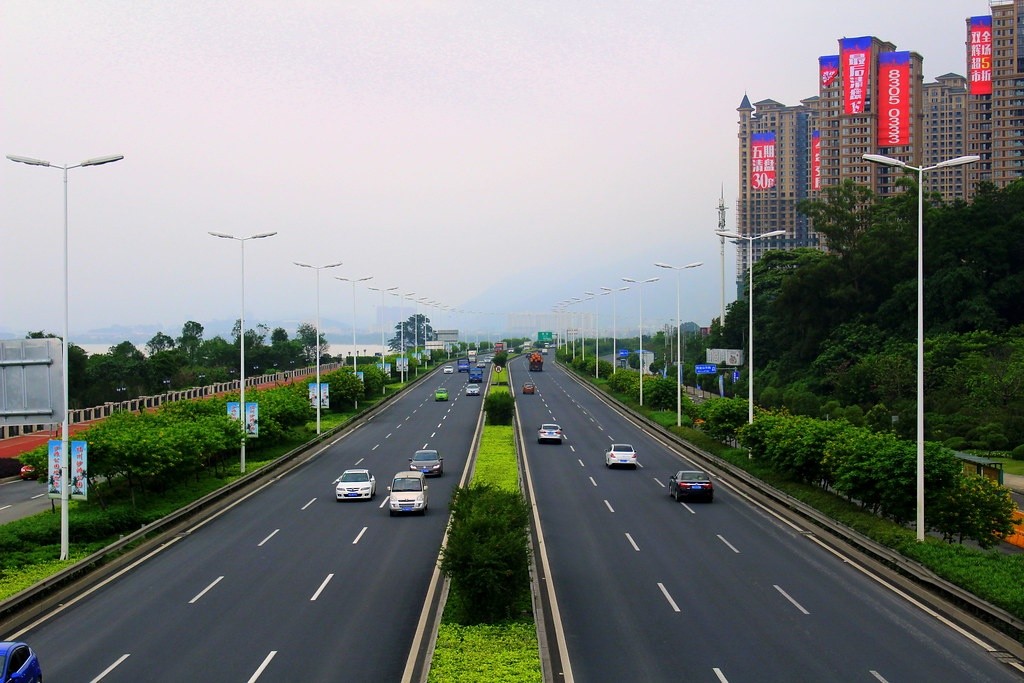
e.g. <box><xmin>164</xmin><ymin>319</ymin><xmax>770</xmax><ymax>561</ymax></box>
<box><xmin>469</xmin><ymin>368</ymin><xmax>484</xmax><ymax>383</ymax></box>
<box><xmin>494</xmin><ymin>342</ymin><xmax>508</xmax><ymax>355</ymax></box>
<box><xmin>529</xmin><ymin>361</ymin><xmax>543</xmax><ymax>372</ymax></box>
<box><xmin>523</xmin><ymin>341</ymin><xmax>534</xmax><ymax>351</ymax></box>
<box><xmin>468</xmin><ymin>351</ymin><xmax>479</xmax><ymax>362</ymax></box>
<box><xmin>457</xmin><ymin>358</ymin><xmax>471</xmax><ymax>373</ymax></box>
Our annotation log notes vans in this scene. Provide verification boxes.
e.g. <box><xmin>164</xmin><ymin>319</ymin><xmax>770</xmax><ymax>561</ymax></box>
<box><xmin>387</xmin><ymin>471</ymin><xmax>434</xmax><ymax>514</ymax></box>
<box><xmin>538</xmin><ymin>343</ymin><xmax>555</xmax><ymax>355</ymax></box>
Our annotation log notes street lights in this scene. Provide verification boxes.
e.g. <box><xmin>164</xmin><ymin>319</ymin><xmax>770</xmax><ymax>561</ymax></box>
<box><xmin>716</xmin><ymin>230</ymin><xmax>790</xmax><ymax>461</ymax></box>
<box><xmin>366</xmin><ymin>285</ymin><xmax>401</xmax><ymax>397</ymax></box>
<box><xmin>862</xmin><ymin>150</ymin><xmax>983</xmax><ymax>546</ymax></box>
<box><xmin>585</xmin><ymin>291</ymin><xmax>611</xmax><ymax>378</ymax></box>
<box><xmin>6</xmin><ymin>152</ymin><xmax>126</xmax><ymax>566</ymax></box>
<box><xmin>390</xmin><ymin>290</ymin><xmax>431</xmax><ymax>383</ymax></box>
<box><xmin>164</xmin><ymin>378</ymin><xmax>172</xmax><ymax>395</ymax></box>
<box><xmin>207</xmin><ymin>229</ymin><xmax>275</xmax><ymax>471</ymax></box>
<box><xmin>600</xmin><ymin>286</ymin><xmax>627</xmax><ymax>375</ymax></box>
<box><xmin>553</xmin><ymin>296</ymin><xmax>593</xmax><ymax>365</ymax></box>
<box><xmin>199</xmin><ymin>373</ymin><xmax>206</xmax><ymax>387</ymax></box>
<box><xmin>654</xmin><ymin>262</ymin><xmax>703</xmax><ymax>426</ymax></box>
<box><xmin>293</xmin><ymin>260</ymin><xmax>340</xmax><ymax>435</ymax></box>
<box><xmin>331</xmin><ymin>273</ymin><xmax>374</xmax><ymax>408</ymax></box>
<box><xmin>116</xmin><ymin>387</ymin><xmax>126</xmax><ymax>403</ymax></box>
<box><xmin>621</xmin><ymin>277</ymin><xmax>661</xmax><ymax>406</ymax></box>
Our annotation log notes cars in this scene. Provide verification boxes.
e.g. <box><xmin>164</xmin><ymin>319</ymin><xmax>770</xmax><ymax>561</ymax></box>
<box><xmin>335</xmin><ymin>469</ymin><xmax>377</xmax><ymax>501</ymax></box>
<box><xmin>484</xmin><ymin>354</ymin><xmax>495</xmax><ymax>363</ymax></box>
<box><xmin>509</xmin><ymin>348</ymin><xmax>515</xmax><ymax>353</ymax></box>
<box><xmin>409</xmin><ymin>449</ymin><xmax>445</xmax><ymax>474</ymax></box>
<box><xmin>604</xmin><ymin>444</ymin><xmax>637</xmax><ymax>470</ymax></box>
<box><xmin>668</xmin><ymin>470</ymin><xmax>715</xmax><ymax>503</ymax></box>
<box><xmin>476</xmin><ymin>361</ymin><xmax>486</xmax><ymax>368</ymax></box>
<box><xmin>537</xmin><ymin>424</ymin><xmax>564</xmax><ymax>444</ymax></box>
<box><xmin>0</xmin><ymin>641</ymin><xmax>43</xmax><ymax>683</ymax></box>
<box><xmin>435</xmin><ymin>388</ymin><xmax>448</xmax><ymax>401</ymax></box>
<box><xmin>525</xmin><ymin>353</ymin><xmax>532</xmax><ymax>359</ymax></box>
<box><xmin>19</xmin><ymin>464</ymin><xmax>48</xmax><ymax>481</ymax></box>
<box><xmin>443</xmin><ymin>366</ymin><xmax>454</xmax><ymax>373</ymax></box>
<box><xmin>465</xmin><ymin>384</ymin><xmax>481</xmax><ymax>396</ymax></box>
<box><xmin>522</xmin><ymin>382</ymin><xmax>536</xmax><ymax>395</ymax></box>
